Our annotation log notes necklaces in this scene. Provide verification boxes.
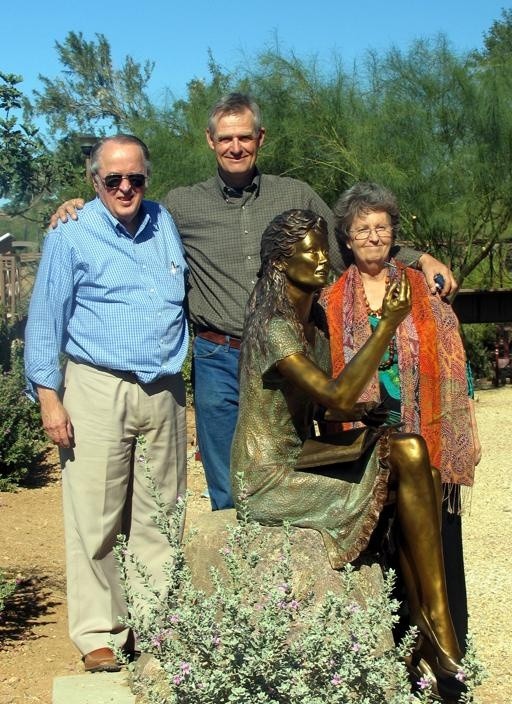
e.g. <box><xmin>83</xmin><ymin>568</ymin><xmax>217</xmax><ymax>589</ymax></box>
<box><xmin>359</xmin><ymin>266</ymin><xmax>391</xmax><ymax>315</ymax></box>
<box><xmin>378</xmin><ymin>338</ymin><xmax>396</xmax><ymax>370</ymax></box>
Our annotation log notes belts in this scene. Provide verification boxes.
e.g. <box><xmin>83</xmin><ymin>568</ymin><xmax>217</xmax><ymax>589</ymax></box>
<box><xmin>193</xmin><ymin>326</ymin><xmax>240</xmax><ymax>349</ymax></box>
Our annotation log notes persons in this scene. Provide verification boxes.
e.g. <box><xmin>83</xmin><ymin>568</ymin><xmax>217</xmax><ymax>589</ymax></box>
<box><xmin>21</xmin><ymin>132</ymin><xmax>189</xmax><ymax>672</ymax></box>
<box><xmin>229</xmin><ymin>208</ymin><xmax>472</xmax><ymax>704</ymax></box>
<box><xmin>50</xmin><ymin>91</ymin><xmax>458</xmax><ymax>512</ymax></box>
<box><xmin>317</xmin><ymin>182</ymin><xmax>482</xmax><ymax>660</ymax></box>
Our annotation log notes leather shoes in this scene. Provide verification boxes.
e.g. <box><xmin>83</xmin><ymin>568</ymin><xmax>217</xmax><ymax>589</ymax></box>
<box><xmin>85</xmin><ymin>648</ymin><xmax>121</xmax><ymax>671</ymax></box>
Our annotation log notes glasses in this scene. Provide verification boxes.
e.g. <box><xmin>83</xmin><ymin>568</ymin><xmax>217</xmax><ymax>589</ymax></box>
<box><xmin>98</xmin><ymin>174</ymin><xmax>147</xmax><ymax>187</ymax></box>
<box><xmin>351</xmin><ymin>225</ymin><xmax>392</xmax><ymax>239</ymax></box>
